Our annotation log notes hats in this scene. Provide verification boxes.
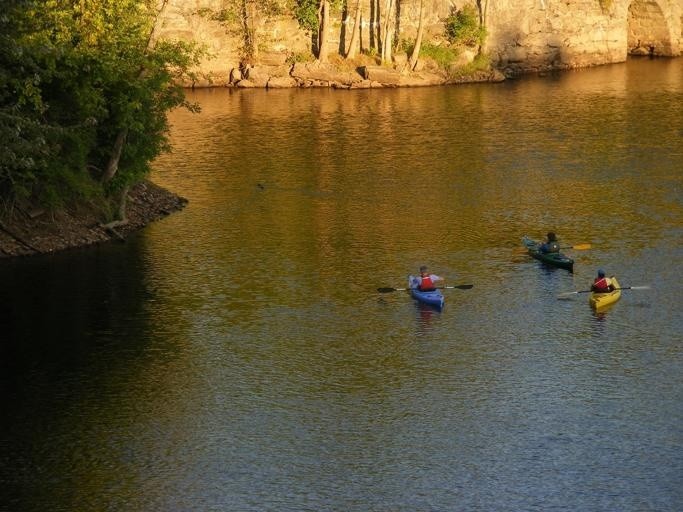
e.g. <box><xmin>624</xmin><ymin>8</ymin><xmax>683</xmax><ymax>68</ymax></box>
<box><xmin>599</xmin><ymin>270</ymin><xmax>605</xmax><ymax>278</ymax></box>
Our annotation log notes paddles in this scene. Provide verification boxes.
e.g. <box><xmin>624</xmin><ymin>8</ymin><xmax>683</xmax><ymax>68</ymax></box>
<box><xmin>557</xmin><ymin>286</ymin><xmax>649</xmax><ymax>298</ymax></box>
<box><xmin>378</xmin><ymin>285</ymin><xmax>473</xmax><ymax>292</ymax></box>
<box><xmin>513</xmin><ymin>243</ymin><xmax>591</xmax><ymax>253</ymax></box>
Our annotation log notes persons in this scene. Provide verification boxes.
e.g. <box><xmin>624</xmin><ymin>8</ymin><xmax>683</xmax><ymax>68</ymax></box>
<box><xmin>535</xmin><ymin>231</ymin><xmax>559</xmax><ymax>254</ymax></box>
<box><xmin>589</xmin><ymin>268</ymin><xmax>617</xmax><ymax>293</ymax></box>
<box><xmin>408</xmin><ymin>265</ymin><xmax>445</xmax><ymax>292</ymax></box>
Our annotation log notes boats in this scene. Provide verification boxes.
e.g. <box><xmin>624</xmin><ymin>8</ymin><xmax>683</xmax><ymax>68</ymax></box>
<box><xmin>590</xmin><ymin>275</ymin><xmax>622</xmax><ymax>312</ymax></box>
<box><xmin>409</xmin><ymin>274</ymin><xmax>444</xmax><ymax>310</ymax></box>
<box><xmin>523</xmin><ymin>237</ymin><xmax>575</xmax><ymax>269</ymax></box>
